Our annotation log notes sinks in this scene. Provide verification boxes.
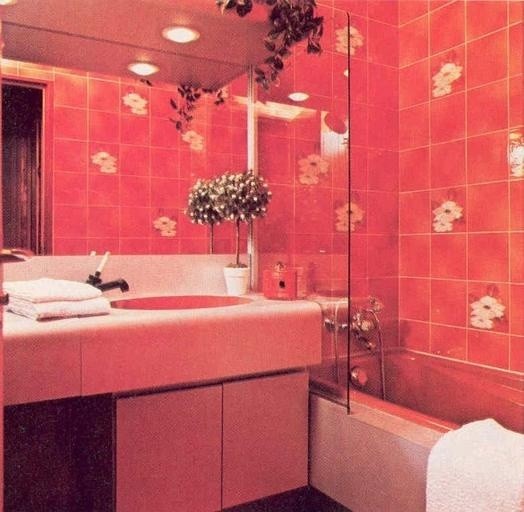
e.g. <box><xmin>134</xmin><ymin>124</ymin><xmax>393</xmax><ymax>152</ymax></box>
<box><xmin>108</xmin><ymin>294</ymin><xmax>253</xmax><ymax>314</ymax></box>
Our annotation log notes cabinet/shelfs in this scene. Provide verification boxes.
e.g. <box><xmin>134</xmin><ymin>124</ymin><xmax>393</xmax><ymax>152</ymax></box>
<box><xmin>114</xmin><ymin>368</ymin><xmax>311</xmax><ymax>512</ymax></box>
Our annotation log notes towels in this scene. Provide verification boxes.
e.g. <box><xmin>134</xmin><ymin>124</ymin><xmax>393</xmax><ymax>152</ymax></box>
<box><xmin>9</xmin><ymin>298</ymin><xmax>111</xmax><ymax>322</ymax></box>
<box><xmin>426</xmin><ymin>413</ymin><xmax>524</xmax><ymax>512</ymax></box>
<box><xmin>6</xmin><ymin>278</ymin><xmax>107</xmax><ymax>304</ymax></box>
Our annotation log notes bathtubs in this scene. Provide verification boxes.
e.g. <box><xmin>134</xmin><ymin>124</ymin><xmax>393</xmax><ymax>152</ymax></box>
<box><xmin>309</xmin><ymin>345</ymin><xmax>523</xmax><ymax>510</ymax></box>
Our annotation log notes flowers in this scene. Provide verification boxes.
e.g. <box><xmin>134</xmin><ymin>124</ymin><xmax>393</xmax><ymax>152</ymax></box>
<box><xmin>185</xmin><ymin>176</ymin><xmax>225</xmax><ymax>255</ymax></box>
<box><xmin>211</xmin><ymin>168</ymin><xmax>273</xmax><ymax>265</ymax></box>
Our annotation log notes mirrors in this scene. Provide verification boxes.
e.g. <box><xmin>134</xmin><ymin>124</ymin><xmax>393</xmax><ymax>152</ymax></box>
<box><xmin>0</xmin><ymin>20</ymin><xmax>257</xmax><ymax>260</ymax></box>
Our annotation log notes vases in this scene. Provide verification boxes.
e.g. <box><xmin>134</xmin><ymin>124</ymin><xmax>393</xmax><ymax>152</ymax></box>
<box><xmin>222</xmin><ymin>267</ymin><xmax>250</xmax><ymax>295</ymax></box>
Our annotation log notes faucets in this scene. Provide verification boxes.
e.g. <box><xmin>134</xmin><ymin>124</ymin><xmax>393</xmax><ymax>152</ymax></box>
<box><xmin>323</xmin><ymin>312</ymin><xmax>389</xmax><ymax>350</ymax></box>
<box><xmin>86</xmin><ymin>271</ymin><xmax>129</xmax><ymax>295</ymax></box>
